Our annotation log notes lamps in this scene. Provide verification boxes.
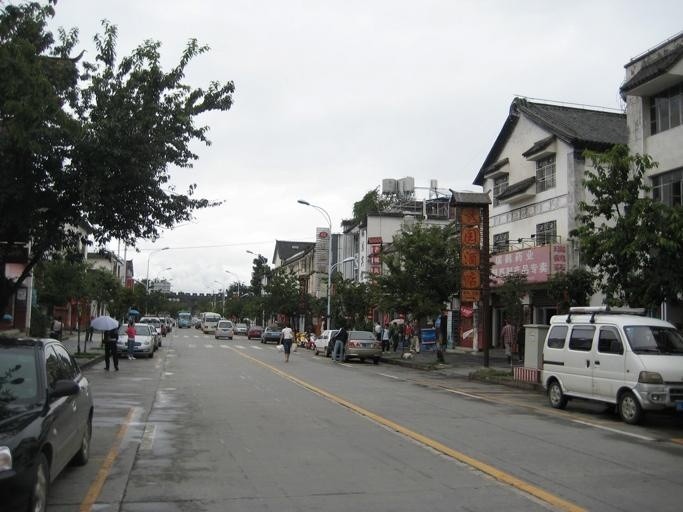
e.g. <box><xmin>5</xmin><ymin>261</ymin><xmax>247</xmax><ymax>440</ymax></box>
<box><xmin>493</xmin><ymin>233</ymin><xmax>561</xmax><ymax>253</ymax></box>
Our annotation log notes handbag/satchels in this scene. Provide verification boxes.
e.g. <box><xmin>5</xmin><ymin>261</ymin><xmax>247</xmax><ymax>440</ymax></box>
<box><xmin>277</xmin><ymin>345</ymin><xmax>284</xmax><ymax>353</ymax></box>
<box><xmin>293</xmin><ymin>343</ymin><xmax>298</xmax><ymax>352</ymax></box>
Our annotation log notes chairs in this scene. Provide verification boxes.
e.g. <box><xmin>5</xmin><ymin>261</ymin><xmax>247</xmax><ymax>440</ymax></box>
<box><xmin>611</xmin><ymin>341</ymin><xmax>620</xmax><ymax>354</ymax></box>
<box><xmin>599</xmin><ymin>344</ymin><xmax>610</xmax><ymax>353</ymax></box>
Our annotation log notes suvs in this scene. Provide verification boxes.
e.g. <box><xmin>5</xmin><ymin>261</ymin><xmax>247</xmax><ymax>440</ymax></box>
<box><xmin>258</xmin><ymin>325</ymin><xmax>281</xmax><ymax>344</ymax></box>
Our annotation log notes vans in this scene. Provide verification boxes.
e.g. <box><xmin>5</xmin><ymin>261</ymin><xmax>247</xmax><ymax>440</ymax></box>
<box><xmin>213</xmin><ymin>320</ymin><xmax>234</xmax><ymax>340</ymax></box>
<box><xmin>538</xmin><ymin>303</ymin><xmax>682</xmax><ymax>425</ymax></box>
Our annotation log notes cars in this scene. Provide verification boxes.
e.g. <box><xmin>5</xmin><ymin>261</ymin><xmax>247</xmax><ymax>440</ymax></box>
<box><xmin>0</xmin><ymin>336</ymin><xmax>97</xmax><ymax>511</ymax></box>
<box><xmin>114</xmin><ymin>323</ymin><xmax>159</xmax><ymax>358</ymax></box>
<box><xmin>246</xmin><ymin>325</ymin><xmax>264</xmax><ymax>340</ymax></box>
<box><xmin>139</xmin><ymin>312</ymin><xmax>202</xmax><ymax>346</ymax></box>
<box><xmin>344</xmin><ymin>330</ymin><xmax>382</xmax><ymax>366</ymax></box>
<box><xmin>233</xmin><ymin>322</ymin><xmax>246</xmax><ymax>336</ymax></box>
<box><xmin>311</xmin><ymin>329</ymin><xmax>340</xmax><ymax>358</ymax></box>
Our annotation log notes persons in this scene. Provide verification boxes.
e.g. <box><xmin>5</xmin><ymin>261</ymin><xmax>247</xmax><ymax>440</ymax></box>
<box><xmin>380</xmin><ymin>324</ymin><xmax>391</xmax><ymax>353</ymax></box>
<box><xmin>103</xmin><ymin>329</ymin><xmax>119</xmax><ymax>370</ymax></box>
<box><xmin>51</xmin><ymin>317</ymin><xmax>56</xmax><ymax>332</ymax></box>
<box><xmin>499</xmin><ymin>320</ymin><xmax>514</xmax><ymax>365</ymax></box>
<box><xmin>389</xmin><ymin>322</ymin><xmax>398</xmax><ymax>352</ymax></box>
<box><xmin>52</xmin><ymin>316</ymin><xmax>63</xmax><ymax>340</ymax></box>
<box><xmin>2</xmin><ymin>307</ymin><xmax>12</xmax><ymax>320</ymax></box>
<box><xmin>278</xmin><ymin>324</ymin><xmax>293</xmax><ymax>362</ymax></box>
<box><xmin>85</xmin><ymin>315</ymin><xmax>94</xmax><ymax>342</ymax></box>
<box><xmin>374</xmin><ymin>322</ymin><xmax>380</xmax><ymax>340</ymax></box>
<box><xmin>125</xmin><ymin>321</ymin><xmax>136</xmax><ymax>360</ymax></box>
<box><xmin>409</xmin><ymin>319</ymin><xmax>420</xmax><ymax>353</ymax></box>
<box><xmin>127</xmin><ymin>314</ymin><xmax>131</xmax><ymax>323</ymax></box>
<box><xmin>331</xmin><ymin>327</ymin><xmax>349</xmax><ymax>363</ymax></box>
<box><xmin>134</xmin><ymin>316</ymin><xmax>138</xmax><ymax>323</ymax></box>
<box><xmin>403</xmin><ymin>323</ymin><xmax>411</xmax><ymax>345</ymax></box>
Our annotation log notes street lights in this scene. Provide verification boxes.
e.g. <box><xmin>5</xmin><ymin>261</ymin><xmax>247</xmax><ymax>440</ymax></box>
<box><xmin>128</xmin><ymin>276</ymin><xmax>147</xmax><ymax>315</ymax></box>
<box><xmin>212</xmin><ymin>279</ymin><xmax>224</xmax><ymax>318</ymax></box>
<box><xmin>224</xmin><ymin>270</ymin><xmax>239</xmax><ymax>296</ymax></box>
<box><xmin>144</xmin><ymin>245</ymin><xmax>172</xmax><ymax>315</ymax></box>
<box><xmin>156</xmin><ymin>266</ymin><xmax>173</xmax><ymax>280</ymax></box>
<box><xmin>326</xmin><ymin>256</ymin><xmax>356</xmax><ymax>329</ymax></box>
<box><xmin>296</xmin><ymin>199</ymin><xmax>334</xmax><ymax>330</ymax></box>
<box><xmin>245</xmin><ymin>249</ymin><xmax>265</xmax><ymax>327</ymax></box>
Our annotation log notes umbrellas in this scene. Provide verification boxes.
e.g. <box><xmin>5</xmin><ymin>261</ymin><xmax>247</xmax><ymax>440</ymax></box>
<box><xmin>89</xmin><ymin>316</ymin><xmax>118</xmax><ymax>331</ymax></box>
<box><xmin>128</xmin><ymin>310</ymin><xmax>140</xmax><ymax>317</ymax></box>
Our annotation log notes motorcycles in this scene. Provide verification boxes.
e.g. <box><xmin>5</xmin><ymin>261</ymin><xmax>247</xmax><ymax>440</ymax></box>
<box><xmin>304</xmin><ymin>330</ymin><xmax>316</xmax><ymax>350</ymax></box>
<box><xmin>295</xmin><ymin>331</ymin><xmax>308</xmax><ymax>347</ymax></box>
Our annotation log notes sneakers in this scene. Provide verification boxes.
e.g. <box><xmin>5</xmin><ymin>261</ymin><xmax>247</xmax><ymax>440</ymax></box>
<box><xmin>132</xmin><ymin>357</ymin><xmax>136</xmax><ymax>359</ymax></box>
<box><xmin>128</xmin><ymin>356</ymin><xmax>133</xmax><ymax>359</ymax></box>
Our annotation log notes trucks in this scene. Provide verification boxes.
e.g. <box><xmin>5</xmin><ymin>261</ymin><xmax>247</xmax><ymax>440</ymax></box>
<box><xmin>199</xmin><ymin>311</ymin><xmax>220</xmax><ymax>335</ymax></box>
